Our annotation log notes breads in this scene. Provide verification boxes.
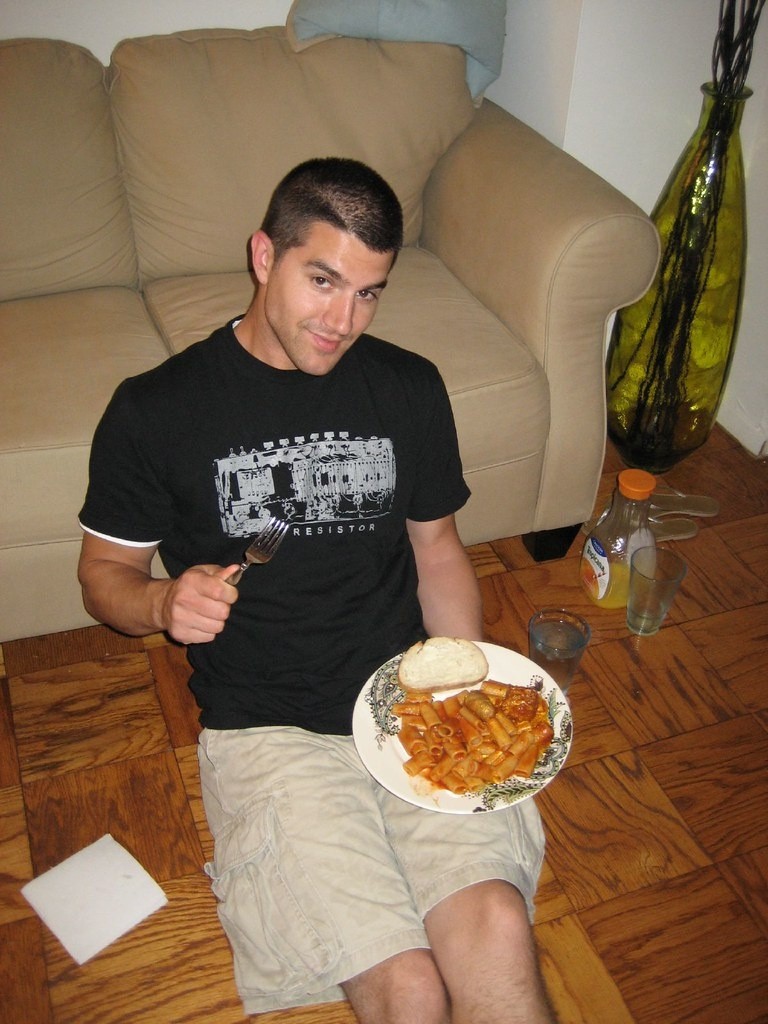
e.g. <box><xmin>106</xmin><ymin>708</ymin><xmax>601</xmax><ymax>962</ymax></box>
<box><xmin>398</xmin><ymin>636</ymin><xmax>489</xmax><ymax>694</ymax></box>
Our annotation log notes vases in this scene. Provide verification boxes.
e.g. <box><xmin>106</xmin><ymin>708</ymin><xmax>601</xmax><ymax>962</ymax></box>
<box><xmin>607</xmin><ymin>82</ymin><xmax>754</xmax><ymax>474</ymax></box>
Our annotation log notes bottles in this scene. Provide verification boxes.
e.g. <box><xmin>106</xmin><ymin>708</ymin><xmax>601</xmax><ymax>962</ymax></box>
<box><xmin>579</xmin><ymin>469</ymin><xmax>658</xmax><ymax>608</ymax></box>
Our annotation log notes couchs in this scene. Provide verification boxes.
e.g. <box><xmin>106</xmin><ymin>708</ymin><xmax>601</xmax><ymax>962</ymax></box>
<box><xmin>0</xmin><ymin>27</ymin><xmax>662</xmax><ymax>648</ymax></box>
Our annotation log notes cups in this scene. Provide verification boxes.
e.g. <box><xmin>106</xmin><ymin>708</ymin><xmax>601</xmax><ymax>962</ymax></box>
<box><xmin>626</xmin><ymin>545</ymin><xmax>688</xmax><ymax>636</ymax></box>
<box><xmin>528</xmin><ymin>608</ymin><xmax>591</xmax><ymax>698</ymax></box>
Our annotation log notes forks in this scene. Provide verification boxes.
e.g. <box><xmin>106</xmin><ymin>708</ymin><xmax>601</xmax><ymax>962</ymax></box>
<box><xmin>223</xmin><ymin>517</ymin><xmax>289</xmax><ymax>586</ymax></box>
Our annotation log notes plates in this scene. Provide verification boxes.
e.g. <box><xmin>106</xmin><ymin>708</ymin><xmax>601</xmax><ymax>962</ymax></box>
<box><xmin>352</xmin><ymin>641</ymin><xmax>573</xmax><ymax>815</ymax></box>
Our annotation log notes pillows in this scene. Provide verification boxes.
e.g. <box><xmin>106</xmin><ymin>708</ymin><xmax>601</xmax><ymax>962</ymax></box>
<box><xmin>284</xmin><ymin>1</ymin><xmax>507</xmax><ymax>109</ymax></box>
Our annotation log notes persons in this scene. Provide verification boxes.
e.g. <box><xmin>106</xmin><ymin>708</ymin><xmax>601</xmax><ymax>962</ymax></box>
<box><xmin>77</xmin><ymin>156</ymin><xmax>545</xmax><ymax>1024</ymax></box>
